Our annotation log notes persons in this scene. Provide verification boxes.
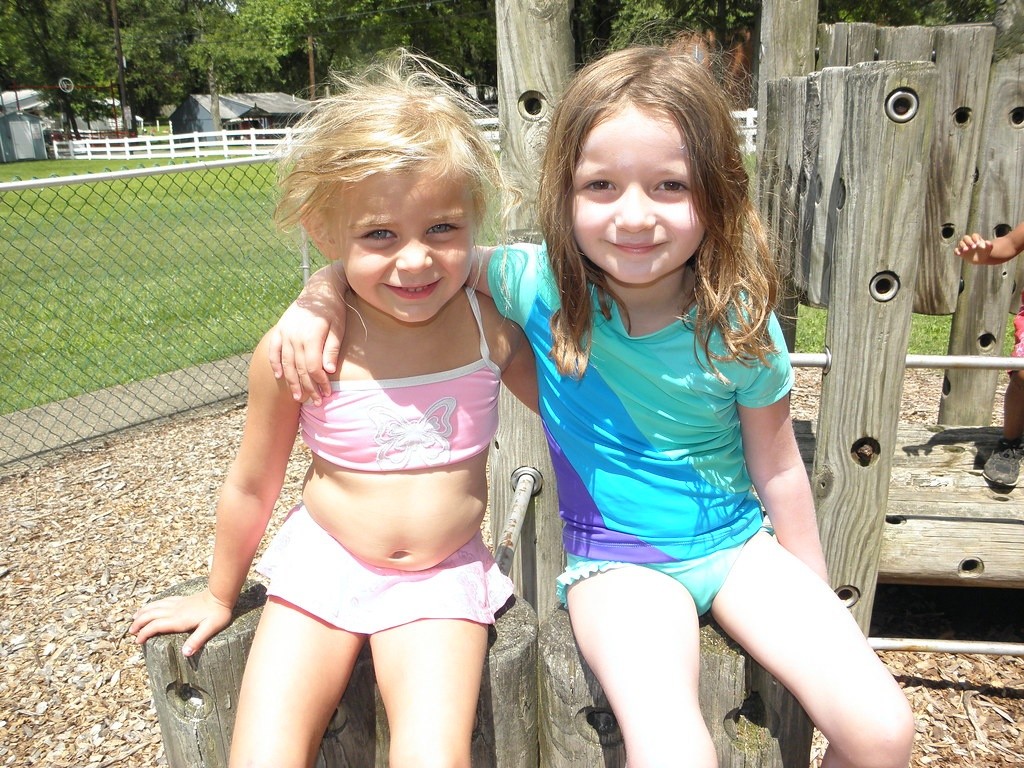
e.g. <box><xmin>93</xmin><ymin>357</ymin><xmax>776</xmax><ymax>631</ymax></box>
<box><xmin>273</xmin><ymin>44</ymin><xmax>909</xmax><ymax>768</ymax></box>
<box><xmin>952</xmin><ymin>222</ymin><xmax>1024</xmax><ymax>487</ymax></box>
<box><xmin>126</xmin><ymin>81</ymin><xmax>544</xmax><ymax>767</ymax></box>
